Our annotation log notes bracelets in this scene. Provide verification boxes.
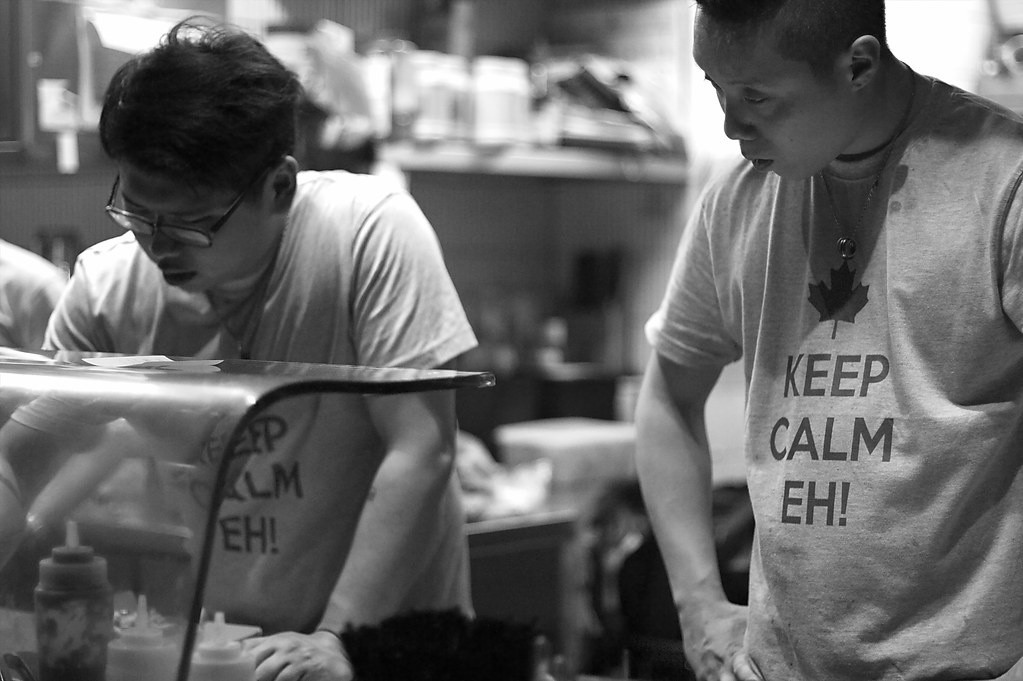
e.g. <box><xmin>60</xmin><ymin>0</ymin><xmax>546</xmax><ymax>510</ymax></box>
<box><xmin>26</xmin><ymin>512</ymin><xmax>44</xmax><ymax>538</ymax></box>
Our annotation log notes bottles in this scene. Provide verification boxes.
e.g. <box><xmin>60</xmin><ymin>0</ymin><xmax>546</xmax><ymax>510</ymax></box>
<box><xmin>33</xmin><ymin>521</ymin><xmax>116</xmax><ymax>681</ymax></box>
<box><xmin>104</xmin><ymin>593</ymin><xmax>182</xmax><ymax>681</ymax></box>
<box><xmin>186</xmin><ymin>612</ymin><xmax>256</xmax><ymax>681</ymax></box>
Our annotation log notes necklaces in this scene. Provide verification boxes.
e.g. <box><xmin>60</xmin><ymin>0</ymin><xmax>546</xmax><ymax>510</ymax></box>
<box><xmin>819</xmin><ymin>62</ymin><xmax>916</xmax><ymax>259</ymax></box>
<box><xmin>207</xmin><ymin>234</ymin><xmax>283</xmax><ymax>359</ymax></box>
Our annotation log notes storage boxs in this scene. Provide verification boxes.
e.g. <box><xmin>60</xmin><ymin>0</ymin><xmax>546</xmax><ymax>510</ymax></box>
<box><xmin>492</xmin><ymin>414</ymin><xmax>636</xmax><ymax>510</ymax></box>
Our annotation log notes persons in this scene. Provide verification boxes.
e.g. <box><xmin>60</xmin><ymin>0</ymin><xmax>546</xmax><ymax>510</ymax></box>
<box><xmin>0</xmin><ymin>238</ymin><xmax>140</xmax><ymax>611</ymax></box>
<box><xmin>634</xmin><ymin>0</ymin><xmax>1023</xmax><ymax>681</ymax></box>
<box><xmin>0</xmin><ymin>16</ymin><xmax>479</xmax><ymax>681</ymax></box>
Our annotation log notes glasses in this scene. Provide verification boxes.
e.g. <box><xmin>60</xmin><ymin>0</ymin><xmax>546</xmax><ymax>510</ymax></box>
<box><xmin>106</xmin><ymin>172</ymin><xmax>257</xmax><ymax>250</ymax></box>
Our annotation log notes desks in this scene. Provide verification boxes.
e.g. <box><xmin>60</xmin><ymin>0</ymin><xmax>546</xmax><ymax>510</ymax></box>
<box><xmin>462</xmin><ymin>509</ymin><xmax>576</xmax><ymax>557</ymax></box>
